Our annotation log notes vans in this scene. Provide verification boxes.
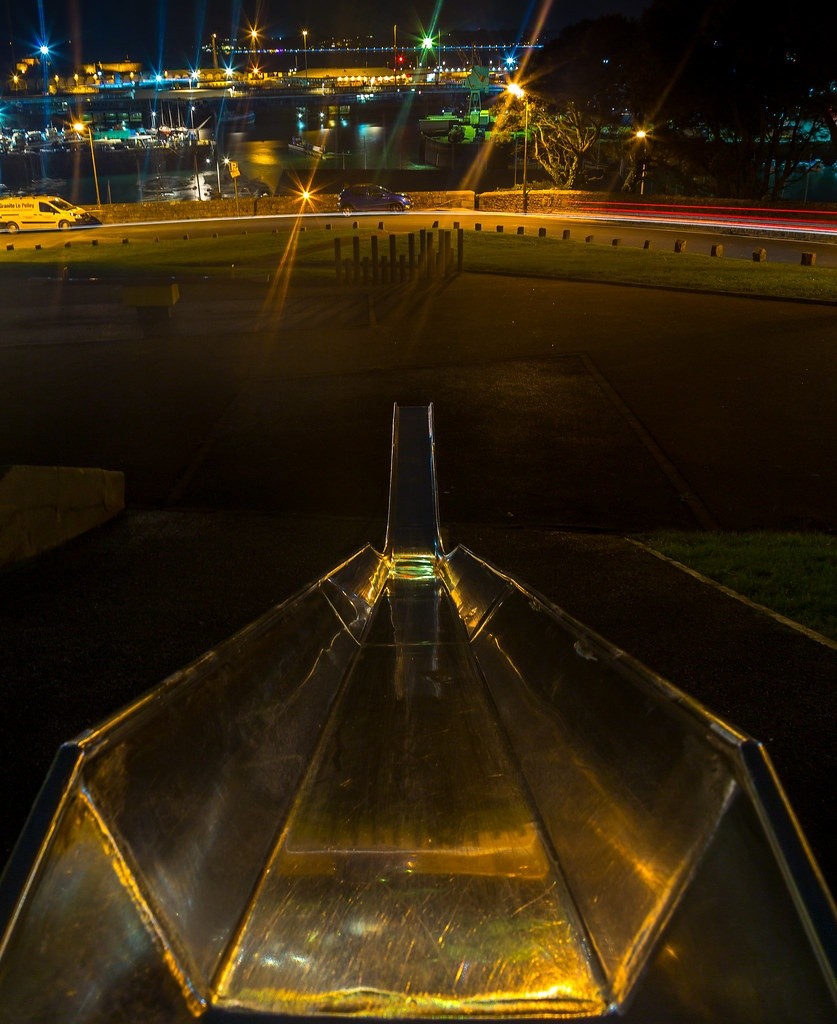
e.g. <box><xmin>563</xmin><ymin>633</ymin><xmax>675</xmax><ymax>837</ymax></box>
<box><xmin>0</xmin><ymin>195</ymin><xmax>102</xmax><ymax>233</ymax></box>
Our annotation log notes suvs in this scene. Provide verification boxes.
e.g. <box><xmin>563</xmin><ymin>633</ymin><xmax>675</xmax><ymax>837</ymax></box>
<box><xmin>338</xmin><ymin>184</ymin><xmax>414</xmax><ymax>220</ymax></box>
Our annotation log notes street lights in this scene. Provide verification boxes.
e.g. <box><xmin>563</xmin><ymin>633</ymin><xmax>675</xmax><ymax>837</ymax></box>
<box><xmin>636</xmin><ymin>129</ymin><xmax>650</xmax><ymax>197</ymax></box>
<box><xmin>302</xmin><ymin>30</ymin><xmax>308</xmax><ymax>88</ymax></box>
<box><xmin>222</xmin><ymin>157</ymin><xmax>239</xmax><ymax>210</ymax></box>
<box><xmin>211</xmin><ymin>33</ymin><xmax>218</xmax><ymax>68</ymax></box>
<box><xmin>71</xmin><ymin>120</ymin><xmax>100</xmax><ymax>211</ymax></box>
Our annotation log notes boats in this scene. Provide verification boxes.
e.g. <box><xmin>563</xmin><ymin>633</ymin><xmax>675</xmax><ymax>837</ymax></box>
<box><xmin>0</xmin><ymin>105</ymin><xmax>216</xmax><ymax>164</ymax></box>
<box><xmin>138</xmin><ymin>174</ymin><xmax>196</xmax><ymax>193</ymax></box>
<box><xmin>296</xmin><ymin>99</ymin><xmax>352</xmax><ymax>115</ymax></box>
<box><xmin>20</xmin><ymin>177</ymin><xmax>67</xmax><ymax>193</ymax></box>
<box><xmin>289</xmin><ymin>136</ymin><xmax>351</xmax><ymax>160</ymax></box>
<box><xmin>419</xmin><ymin>109</ymin><xmax>511</xmax><ymax>173</ymax></box>
<box><xmin>218</xmin><ymin>106</ymin><xmax>258</xmax><ymax>126</ymax></box>
